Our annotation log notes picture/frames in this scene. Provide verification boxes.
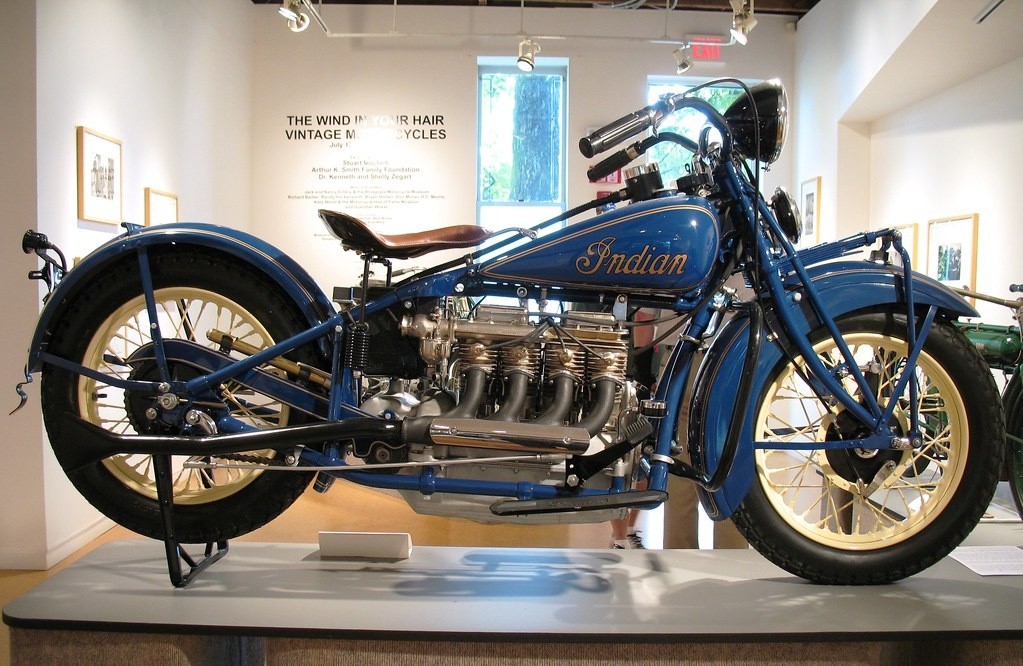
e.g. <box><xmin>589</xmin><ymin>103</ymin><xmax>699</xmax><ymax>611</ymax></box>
<box><xmin>927</xmin><ymin>214</ymin><xmax>979</xmax><ymax>313</ymax></box>
<box><xmin>144</xmin><ymin>187</ymin><xmax>178</xmax><ymax>227</ymax></box>
<box><xmin>76</xmin><ymin>125</ymin><xmax>123</xmax><ymax>225</ymax></box>
<box><xmin>896</xmin><ymin>223</ymin><xmax>918</xmax><ymax>271</ymax></box>
<box><xmin>801</xmin><ymin>176</ymin><xmax>822</xmax><ymax>243</ymax></box>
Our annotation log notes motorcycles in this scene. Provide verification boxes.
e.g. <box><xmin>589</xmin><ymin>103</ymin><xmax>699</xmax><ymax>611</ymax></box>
<box><xmin>8</xmin><ymin>76</ymin><xmax>1007</xmax><ymax>589</ymax></box>
<box><xmin>874</xmin><ymin>285</ymin><xmax>1023</xmax><ymax>520</ymax></box>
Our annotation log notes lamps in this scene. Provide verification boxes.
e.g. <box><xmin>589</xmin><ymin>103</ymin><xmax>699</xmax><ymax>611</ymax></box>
<box><xmin>673</xmin><ymin>45</ymin><xmax>691</xmax><ymax>75</ymax></box>
<box><xmin>279</xmin><ymin>0</ymin><xmax>311</xmax><ymax>33</ymax></box>
<box><xmin>730</xmin><ymin>0</ymin><xmax>758</xmax><ymax>46</ymax></box>
<box><xmin>517</xmin><ymin>37</ymin><xmax>541</xmax><ymax>72</ymax></box>
